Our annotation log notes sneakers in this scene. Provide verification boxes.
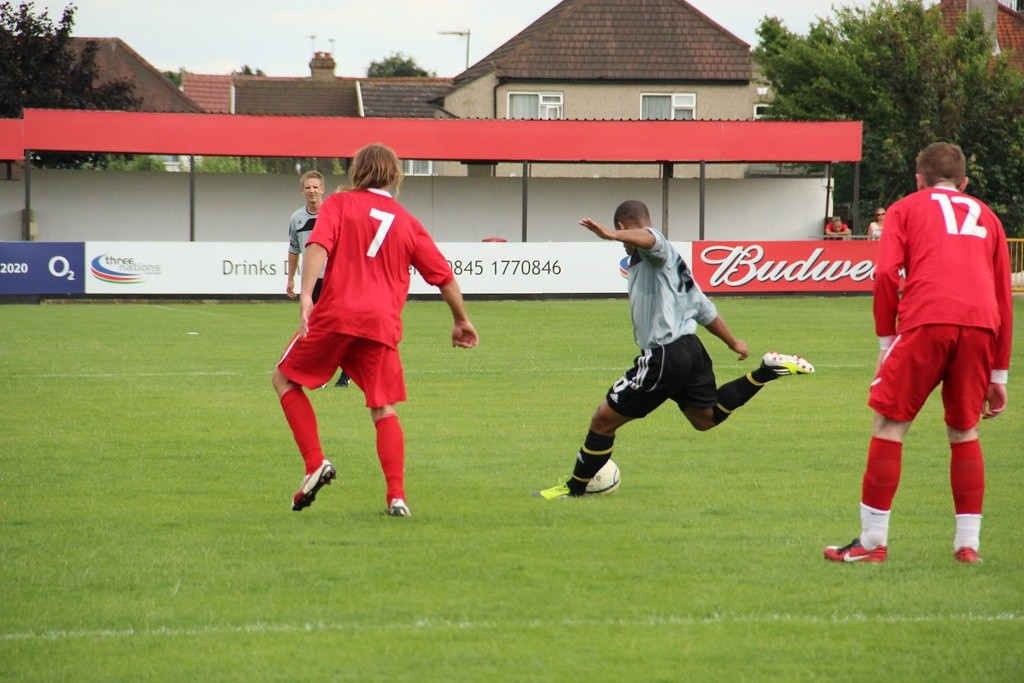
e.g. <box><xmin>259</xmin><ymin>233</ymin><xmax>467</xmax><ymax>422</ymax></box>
<box><xmin>334</xmin><ymin>370</ymin><xmax>352</xmax><ymax>388</ymax></box>
<box><xmin>290</xmin><ymin>458</ymin><xmax>338</xmax><ymax>512</ymax></box>
<box><xmin>955</xmin><ymin>546</ymin><xmax>984</xmax><ymax>564</ymax></box>
<box><xmin>758</xmin><ymin>352</ymin><xmax>816</xmax><ymax>378</ymax></box>
<box><xmin>386</xmin><ymin>497</ymin><xmax>415</xmax><ymax>519</ymax></box>
<box><xmin>531</xmin><ymin>480</ymin><xmax>581</xmax><ymax>503</ymax></box>
<box><xmin>822</xmin><ymin>536</ymin><xmax>888</xmax><ymax>563</ymax></box>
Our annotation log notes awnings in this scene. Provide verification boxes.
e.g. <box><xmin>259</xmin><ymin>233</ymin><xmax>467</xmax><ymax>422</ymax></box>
<box><xmin>20</xmin><ymin>106</ymin><xmax>863</xmax><ymax>241</ymax></box>
<box><xmin>0</xmin><ymin>118</ymin><xmax>24</xmax><ymax>162</ymax></box>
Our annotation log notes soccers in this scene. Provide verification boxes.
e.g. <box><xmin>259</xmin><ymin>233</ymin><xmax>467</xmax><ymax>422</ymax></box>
<box><xmin>586</xmin><ymin>458</ymin><xmax>621</xmax><ymax>495</ymax></box>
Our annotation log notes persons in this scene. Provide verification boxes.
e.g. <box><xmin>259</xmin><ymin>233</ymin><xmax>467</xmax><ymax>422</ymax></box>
<box><xmin>531</xmin><ymin>200</ymin><xmax>816</xmax><ymax>502</ymax></box>
<box><xmin>272</xmin><ymin>143</ymin><xmax>480</xmax><ymax>514</ymax></box>
<box><xmin>286</xmin><ymin>172</ymin><xmax>354</xmax><ymax>388</ymax></box>
<box><xmin>867</xmin><ymin>205</ymin><xmax>887</xmax><ymax>240</ymax></box>
<box><xmin>821</xmin><ymin>140</ymin><xmax>1012</xmax><ymax>564</ymax></box>
<box><xmin>825</xmin><ymin>216</ymin><xmax>849</xmax><ymax>240</ymax></box>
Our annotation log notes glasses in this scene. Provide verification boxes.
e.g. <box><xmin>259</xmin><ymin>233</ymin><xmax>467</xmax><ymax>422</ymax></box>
<box><xmin>876</xmin><ymin>212</ymin><xmax>885</xmax><ymax>217</ymax></box>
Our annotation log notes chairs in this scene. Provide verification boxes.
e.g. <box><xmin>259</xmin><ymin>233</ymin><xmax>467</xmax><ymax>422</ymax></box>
<box><xmin>481</xmin><ymin>238</ymin><xmax>507</xmax><ymax>242</ymax></box>
